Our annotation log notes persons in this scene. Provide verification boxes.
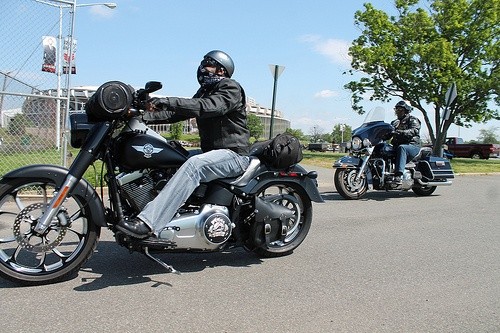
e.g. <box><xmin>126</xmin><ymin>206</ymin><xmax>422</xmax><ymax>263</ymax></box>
<box><xmin>115</xmin><ymin>50</ymin><xmax>250</xmax><ymax>238</ymax></box>
<box><xmin>43</xmin><ymin>38</ymin><xmax>56</xmax><ymax>65</ymax></box>
<box><xmin>387</xmin><ymin>101</ymin><xmax>421</xmax><ymax>181</ymax></box>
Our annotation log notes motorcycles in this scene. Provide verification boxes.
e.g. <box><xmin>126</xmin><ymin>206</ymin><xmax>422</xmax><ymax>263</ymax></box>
<box><xmin>0</xmin><ymin>80</ymin><xmax>326</xmax><ymax>287</ymax></box>
<box><xmin>331</xmin><ymin>105</ymin><xmax>456</xmax><ymax>201</ymax></box>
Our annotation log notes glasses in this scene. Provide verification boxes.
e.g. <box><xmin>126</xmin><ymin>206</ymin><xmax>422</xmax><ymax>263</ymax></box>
<box><xmin>200</xmin><ymin>59</ymin><xmax>213</xmax><ymax>66</ymax></box>
<box><xmin>395</xmin><ymin>108</ymin><xmax>403</xmax><ymax>112</ymax></box>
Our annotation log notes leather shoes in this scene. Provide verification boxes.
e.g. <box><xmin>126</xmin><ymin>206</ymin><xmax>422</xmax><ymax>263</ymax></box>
<box><xmin>117</xmin><ymin>218</ymin><xmax>151</xmax><ymax>238</ymax></box>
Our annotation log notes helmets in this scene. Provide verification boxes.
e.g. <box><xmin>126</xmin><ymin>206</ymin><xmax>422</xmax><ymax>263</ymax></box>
<box><xmin>204</xmin><ymin>50</ymin><xmax>234</xmax><ymax>78</ymax></box>
<box><xmin>395</xmin><ymin>101</ymin><xmax>413</xmax><ymax>112</ymax></box>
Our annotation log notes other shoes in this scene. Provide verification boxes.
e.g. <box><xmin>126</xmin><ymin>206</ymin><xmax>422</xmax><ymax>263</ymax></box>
<box><xmin>390</xmin><ymin>175</ymin><xmax>403</xmax><ymax>188</ymax></box>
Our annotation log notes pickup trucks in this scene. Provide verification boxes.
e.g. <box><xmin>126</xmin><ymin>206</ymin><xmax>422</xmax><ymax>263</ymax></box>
<box><xmin>422</xmin><ymin>136</ymin><xmax>500</xmax><ymax>160</ymax></box>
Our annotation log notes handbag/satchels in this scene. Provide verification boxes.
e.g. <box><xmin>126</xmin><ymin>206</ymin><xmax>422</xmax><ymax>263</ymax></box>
<box><xmin>270</xmin><ymin>133</ymin><xmax>304</xmax><ymax>173</ymax></box>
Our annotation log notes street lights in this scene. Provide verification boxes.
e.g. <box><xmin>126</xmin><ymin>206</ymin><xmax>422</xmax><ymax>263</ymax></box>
<box><xmin>35</xmin><ymin>0</ymin><xmax>117</xmax><ymax>169</ymax></box>
